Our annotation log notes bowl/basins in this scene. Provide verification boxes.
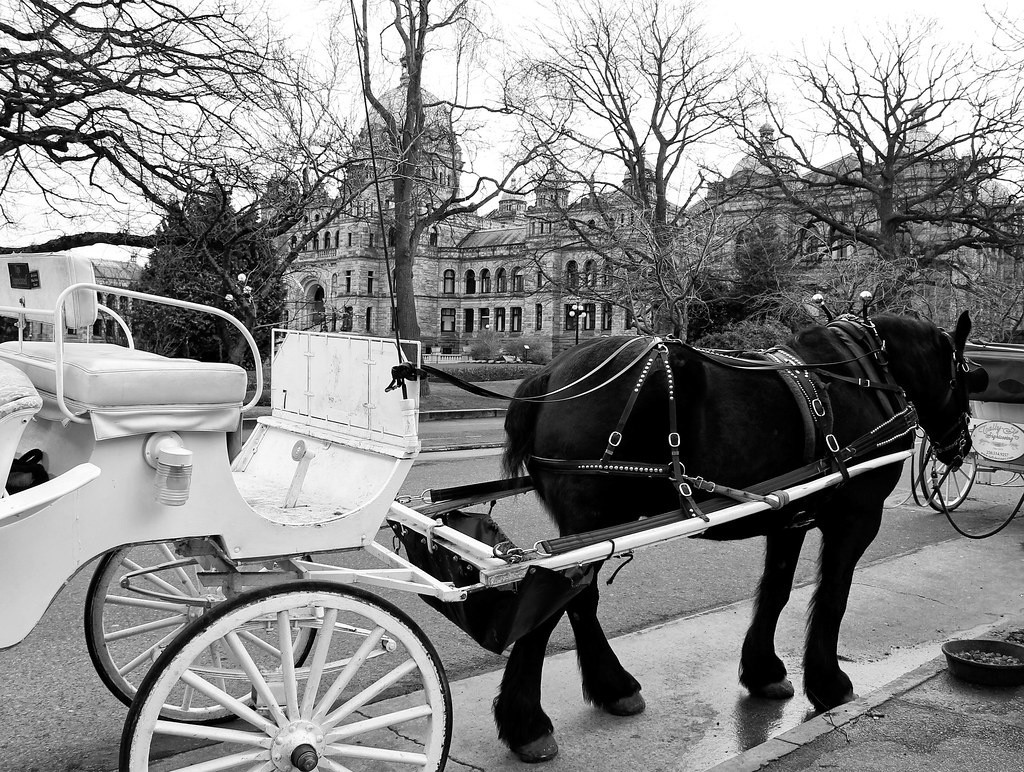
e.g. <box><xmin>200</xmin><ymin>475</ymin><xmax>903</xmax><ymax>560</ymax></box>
<box><xmin>941</xmin><ymin>639</ymin><xmax>1024</xmax><ymax>686</ymax></box>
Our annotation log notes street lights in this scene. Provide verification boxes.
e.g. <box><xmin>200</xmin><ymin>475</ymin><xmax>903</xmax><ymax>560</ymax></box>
<box><xmin>569</xmin><ymin>297</ymin><xmax>586</xmax><ymax>346</ymax></box>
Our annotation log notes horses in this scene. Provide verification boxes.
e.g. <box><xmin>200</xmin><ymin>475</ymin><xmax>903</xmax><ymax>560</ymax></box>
<box><xmin>493</xmin><ymin>308</ymin><xmax>973</xmax><ymax>764</ymax></box>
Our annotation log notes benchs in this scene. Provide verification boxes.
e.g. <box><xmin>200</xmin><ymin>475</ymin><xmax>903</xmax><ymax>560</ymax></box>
<box><xmin>962</xmin><ymin>341</ymin><xmax>1024</xmax><ymax>403</ymax></box>
<box><xmin>0</xmin><ymin>254</ymin><xmax>265</xmax><ymax>436</ymax></box>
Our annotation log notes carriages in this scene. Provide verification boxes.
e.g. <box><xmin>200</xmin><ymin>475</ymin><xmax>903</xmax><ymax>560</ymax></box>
<box><xmin>0</xmin><ymin>253</ymin><xmax>972</xmax><ymax>771</ymax></box>
